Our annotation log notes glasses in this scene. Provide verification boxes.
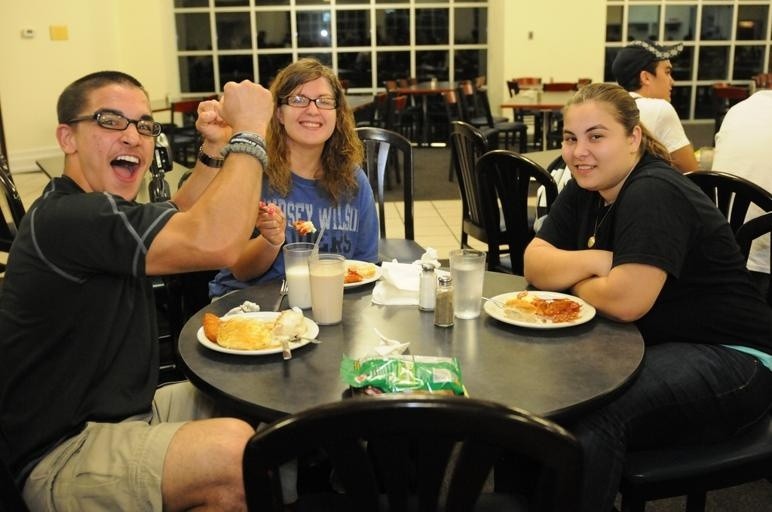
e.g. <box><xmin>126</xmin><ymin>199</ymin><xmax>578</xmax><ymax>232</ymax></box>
<box><xmin>281</xmin><ymin>96</ymin><xmax>339</xmax><ymax>110</ymax></box>
<box><xmin>66</xmin><ymin>112</ymin><xmax>162</xmax><ymax>137</ymax></box>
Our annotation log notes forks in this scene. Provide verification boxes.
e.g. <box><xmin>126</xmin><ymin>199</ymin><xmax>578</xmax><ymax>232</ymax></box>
<box><xmin>272</xmin><ymin>279</ymin><xmax>288</xmax><ymax>311</ymax></box>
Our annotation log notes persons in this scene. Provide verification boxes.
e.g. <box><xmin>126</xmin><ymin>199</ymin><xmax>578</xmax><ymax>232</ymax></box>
<box><xmin>207</xmin><ymin>58</ymin><xmax>380</xmax><ymax>306</ymax></box>
<box><xmin>522</xmin><ymin>82</ymin><xmax>772</xmax><ymax>512</ymax></box>
<box><xmin>607</xmin><ymin>40</ymin><xmax>700</xmax><ymax>176</ymax></box>
<box><xmin>0</xmin><ymin>70</ymin><xmax>275</xmax><ymax>512</ymax></box>
<box><xmin>185</xmin><ymin>18</ymin><xmax>481</xmax><ymax>91</ymax></box>
<box><xmin>606</xmin><ymin>14</ymin><xmax>764</xmax><ymax>79</ymax></box>
<box><xmin>710</xmin><ymin>88</ymin><xmax>772</xmax><ymax>304</ymax></box>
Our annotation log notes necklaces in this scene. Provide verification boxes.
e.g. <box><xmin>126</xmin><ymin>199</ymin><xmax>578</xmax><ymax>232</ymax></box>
<box><xmin>586</xmin><ymin>192</ymin><xmax>617</xmax><ymax>250</ymax></box>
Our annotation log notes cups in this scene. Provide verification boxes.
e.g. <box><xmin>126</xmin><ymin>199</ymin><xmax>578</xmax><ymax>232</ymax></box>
<box><xmin>306</xmin><ymin>253</ymin><xmax>348</xmax><ymax>326</ymax></box>
<box><xmin>282</xmin><ymin>242</ymin><xmax>319</xmax><ymax>310</ymax></box>
<box><xmin>447</xmin><ymin>248</ymin><xmax>486</xmax><ymax>321</ymax></box>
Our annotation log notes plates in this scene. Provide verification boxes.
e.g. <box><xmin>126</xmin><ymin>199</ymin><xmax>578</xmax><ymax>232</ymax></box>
<box><xmin>196</xmin><ymin>310</ymin><xmax>320</xmax><ymax>357</ymax></box>
<box><xmin>340</xmin><ymin>259</ymin><xmax>382</xmax><ymax>289</ymax></box>
<box><xmin>483</xmin><ymin>289</ymin><xmax>596</xmax><ymax>331</ymax></box>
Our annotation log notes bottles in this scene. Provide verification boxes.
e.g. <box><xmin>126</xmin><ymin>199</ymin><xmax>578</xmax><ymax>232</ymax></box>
<box><xmin>417</xmin><ymin>263</ymin><xmax>437</xmax><ymax>312</ymax></box>
<box><xmin>433</xmin><ymin>276</ymin><xmax>455</xmax><ymax>328</ymax></box>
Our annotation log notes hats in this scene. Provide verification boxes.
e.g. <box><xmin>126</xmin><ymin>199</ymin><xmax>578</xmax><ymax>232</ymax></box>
<box><xmin>612</xmin><ymin>40</ymin><xmax>683</xmax><ymax>85</ymax></box>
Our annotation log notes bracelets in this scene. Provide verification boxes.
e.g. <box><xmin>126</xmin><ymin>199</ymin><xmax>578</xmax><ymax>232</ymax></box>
<box><xmin>229</xmin><ymin>132</ymin><xmax>266</xmax><ymax>153</ymax></box>
<box><xmin>198</xmin><ymin>145</ymin><xmax>223</xmax><ymax>169</ymax></box>
<box><xmin>219</xmin><ymin>139</ymin><xmax>271</xmax><ymax>175</ymax></box>
